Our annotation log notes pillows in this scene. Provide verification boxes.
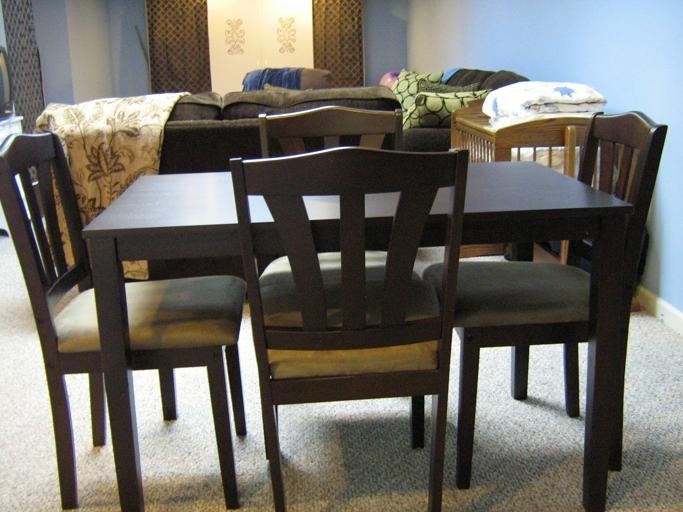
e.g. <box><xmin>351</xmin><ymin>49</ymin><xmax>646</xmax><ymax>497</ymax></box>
<box><xmin>242</xmin><ymin>68</ymin><xmax>332</xmax><ymax>91</ymax></box>
<box><xmin>401</xmin><ymin>89</ymin><xmax>494</xmax><ymax>130</ymax></box>
<box><xmin>416</xmin><ymin>78</ymin><xmax>481</xmax><ymax>95</ymax></box>
<box><xmin>379</xmin><ymin>72</ymin><xmax>399</xmax><ymax>88</ymax></box>
<box><xmin>390</xmin><ymin>67</ymin><xmax>445</xmax><ymax>119</ymax></box>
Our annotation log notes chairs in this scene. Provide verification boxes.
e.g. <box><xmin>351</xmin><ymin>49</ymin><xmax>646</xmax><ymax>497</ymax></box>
<box><xmin>257</xmin><ymin>104</ymin><xmax>402</xmax><ymax>159</ymax></box>
<box><xmin>229</xmin><ymin>146</ymin><xmax>469</xmax><ymax>512</ymax></box>
<box><xmin>419</xmin><ymin>110</ymin><xmax>669</xmax><ymax>493</ymax></box>
<box><xmin>0</xmin><ymin>129</ymin><xmax>248</xmax><ymax>512</ymax></box>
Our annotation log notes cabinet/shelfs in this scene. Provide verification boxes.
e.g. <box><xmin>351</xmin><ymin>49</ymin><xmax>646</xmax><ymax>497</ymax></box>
<box><xmin>0</xmin><ymin>115</ymin><xmax>24</xmax><ymax>239</ymax></box>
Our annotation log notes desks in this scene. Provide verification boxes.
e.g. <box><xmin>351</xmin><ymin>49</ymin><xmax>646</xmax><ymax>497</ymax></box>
<box><xmin>82</xmin><ymin>160</ymin><xmax>633</xmax><ymax>512</ymax></box>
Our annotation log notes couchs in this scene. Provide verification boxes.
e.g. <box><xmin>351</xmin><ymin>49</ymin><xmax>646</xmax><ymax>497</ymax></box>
<box><xmin>34</xmin><ymin>68</ymin><xmax>534</xmax><ymax>280</ymax></box>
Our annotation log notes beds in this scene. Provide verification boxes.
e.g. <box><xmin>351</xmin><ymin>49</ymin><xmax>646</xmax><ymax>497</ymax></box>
<box><xmin>449</xmin><ymin>104</ymin><xmax>604</xmax><ymax>262</ymax></box>
